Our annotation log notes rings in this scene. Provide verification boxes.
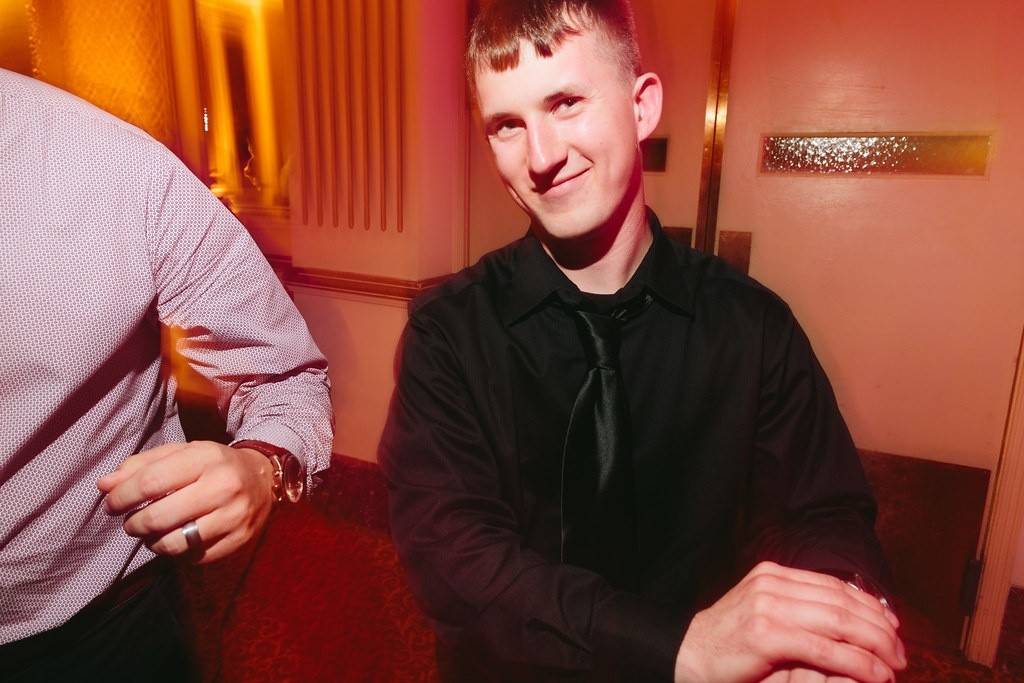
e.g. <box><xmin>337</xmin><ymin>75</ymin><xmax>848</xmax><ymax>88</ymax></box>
<box><xmin>181</xmin><ymin>521</ymin><xmax>205</xmax><ymax>560</ymax></box>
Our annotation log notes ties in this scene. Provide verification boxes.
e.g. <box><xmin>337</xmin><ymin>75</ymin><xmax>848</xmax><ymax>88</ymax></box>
<box><xmin>558</xmin><ymin>301</ymin><xmax>642</xmax><ymax>589</ymax></box>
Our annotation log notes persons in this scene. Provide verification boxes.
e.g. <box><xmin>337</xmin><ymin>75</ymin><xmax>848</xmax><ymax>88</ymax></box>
<box><xmin>378</xmin><ymin>1</ymin><xmax>906</xmax><ymax>683</ymax></box>
<box><xmin>0</xmin><ymin>69</ymin><xmax>334</xmax><ymax>683</ymax></box>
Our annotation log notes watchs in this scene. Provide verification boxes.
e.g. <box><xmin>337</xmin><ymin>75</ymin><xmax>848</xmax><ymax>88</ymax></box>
<box><xmin>233</xmin><ymin>440</ymin><xmax>303</xmax><ymax>506</ymax></box>
